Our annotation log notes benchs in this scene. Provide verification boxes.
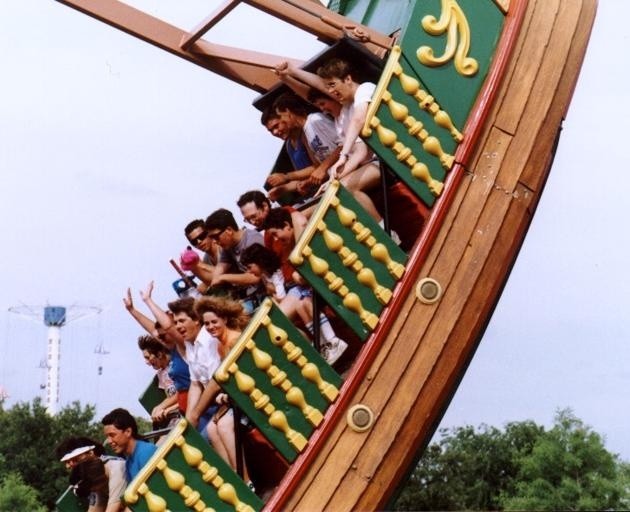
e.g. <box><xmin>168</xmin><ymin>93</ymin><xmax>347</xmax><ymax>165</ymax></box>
<box><xmin>57</xmin><ymin>412</ymin><xmax>278</xmax><ymax>512</ymax></box>
<box><xmin>248</xmin><ymin>35</ymin><xmax>480</xmax><ymax>236</ymax></box>
<box><xmin>136</xmin><ymin>297</ymin><xmax>344</xmax><ymax>483</ymax></box>
<box><xmin>222</xmin><ymin>182</ymin><xmax>410</xmax><ymax>365</ymax></box>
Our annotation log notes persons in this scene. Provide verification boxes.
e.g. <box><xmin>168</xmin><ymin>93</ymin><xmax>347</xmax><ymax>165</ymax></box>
<box><xmin>54</xmin><ymin>31</ymin><xmax>382</xmax><ymax>512</ymax></box>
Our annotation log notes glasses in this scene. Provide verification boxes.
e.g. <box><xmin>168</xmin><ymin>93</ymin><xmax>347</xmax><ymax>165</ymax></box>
<box><xmin>208</xmin><ymin>229</ymin><xmax>224</xmax><ymax>240</ymax></box>
<box><xmin>190</xmin><ymin>231</ymin><xmax>206</xmax><ymax>245</ymax></box>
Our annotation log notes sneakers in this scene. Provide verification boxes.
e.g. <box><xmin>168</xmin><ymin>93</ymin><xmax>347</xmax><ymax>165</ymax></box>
<box><xmin>320</xmin><ymin>339</ymin><xmax>347</xmax><ymax>366</ymax></box>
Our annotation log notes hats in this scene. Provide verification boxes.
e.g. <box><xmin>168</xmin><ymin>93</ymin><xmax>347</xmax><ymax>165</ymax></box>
<box><xmin>60</xmin><ymin>444</ymin><xmax>96</xmax><ymax>462</ymax></box>
